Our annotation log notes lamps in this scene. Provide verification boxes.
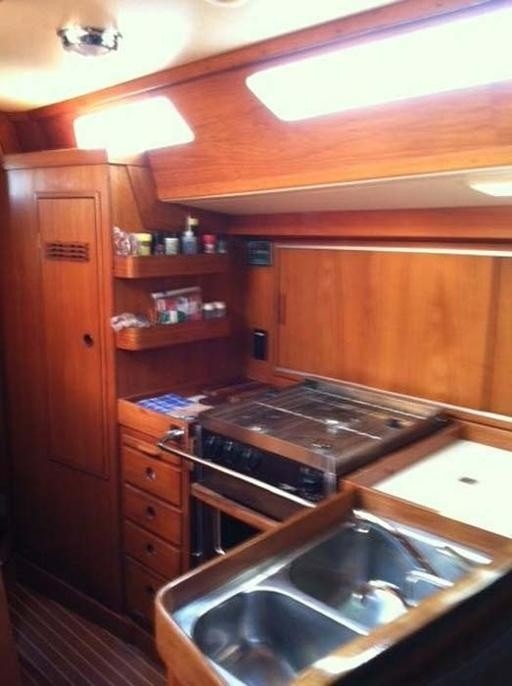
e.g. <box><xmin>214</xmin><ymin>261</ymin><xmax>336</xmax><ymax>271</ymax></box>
<box><xmin>56</xmin><ymin>25</ymin><xmax>122</xmax><ymax>58</ymax></box>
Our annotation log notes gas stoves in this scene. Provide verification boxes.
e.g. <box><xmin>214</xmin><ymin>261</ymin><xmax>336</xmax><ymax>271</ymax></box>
<box><xmin>190</xmin><ymin>380</ymin><xmax>448</xmax><ymax>511</ymax></box>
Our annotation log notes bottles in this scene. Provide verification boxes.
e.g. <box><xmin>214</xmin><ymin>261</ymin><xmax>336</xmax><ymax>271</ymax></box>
<box><xmin>129</xmin><ymin>230</ymin><xmax>226</xmax><ymax>329</ymax></box>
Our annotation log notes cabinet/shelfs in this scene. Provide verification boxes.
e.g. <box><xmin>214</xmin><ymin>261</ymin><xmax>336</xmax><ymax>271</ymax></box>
<box><xmin>39</xmin><ymin>193</ymin><xmax>227</xmax><ymax>479</ymax></box>
<box><xmin>117</xmin><ymin>425</ymin><xmax>184</xmax><ymax>630</ymax></box>
<box><xmin>109</xmin><ymin>246</ymin><xmax>236</xmax><ymax>358</ymax></box>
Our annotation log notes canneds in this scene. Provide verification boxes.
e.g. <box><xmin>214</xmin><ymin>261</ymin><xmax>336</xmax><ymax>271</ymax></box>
<box><xmin>132</xmin><ymin>233</ymin><xmax>152</xmax><ymax>257</ymax></box>
